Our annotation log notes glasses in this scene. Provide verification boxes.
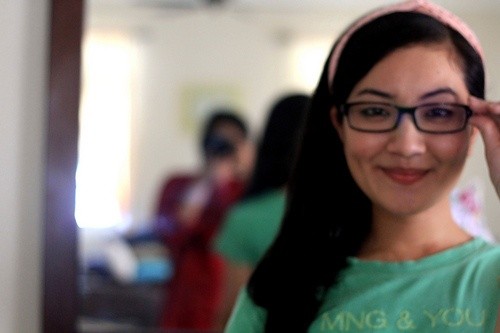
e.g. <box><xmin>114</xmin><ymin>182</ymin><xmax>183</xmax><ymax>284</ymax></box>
<box><xmin>338</xmin><ymin>101</ymin><xmax>475</xmax><ymax>134</ymax></box>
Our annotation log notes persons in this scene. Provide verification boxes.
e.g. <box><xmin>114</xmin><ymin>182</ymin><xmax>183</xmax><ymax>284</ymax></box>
<box><xmin>158</xmin><ymin>110</ymin><xmax>258</xmax><ymax>333</ymax></box>
<box><xmin>224</xmin><ymin>0</ymin><xmax>500</xmax><ymax>333</ymax></box>
<box><xmin>211</xmin><ymin>94</ymin><xmax>312</xmax><ymax>332</ymax></box>
